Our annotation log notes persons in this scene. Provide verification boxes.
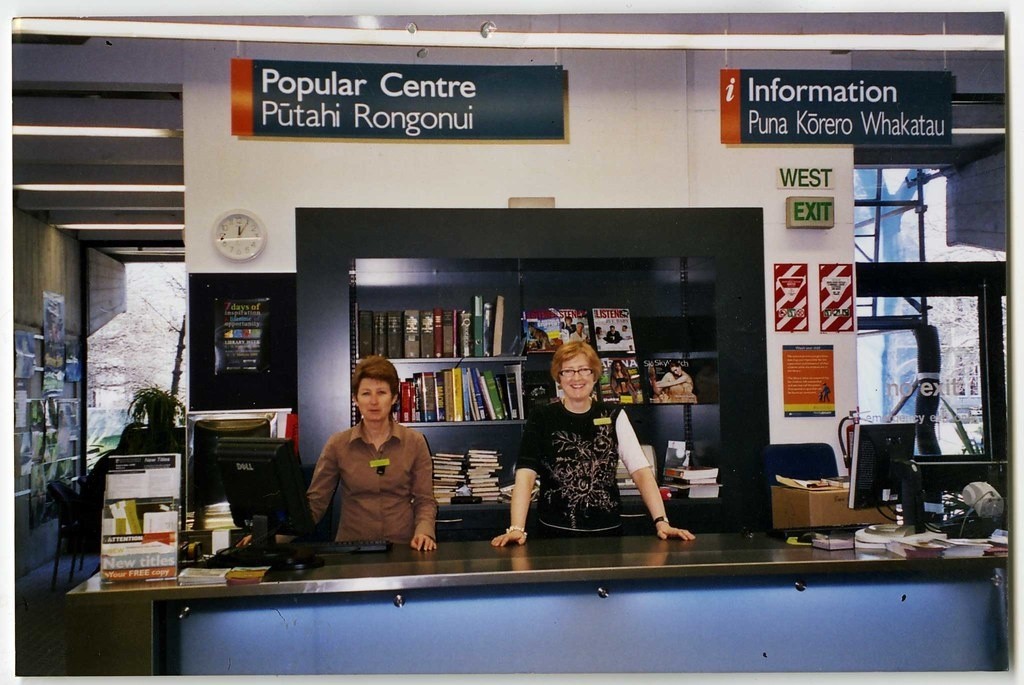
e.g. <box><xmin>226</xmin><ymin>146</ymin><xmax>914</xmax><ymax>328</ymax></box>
<box><xmin>492</xmin><ymin>538</ymin><xmax>691</xmax><ymax>571</ymax></box>
<box><xmin>597</xmin><ymin>325</ymin><xmax>632</xmax><ymax>345</ymax></box>
<box><xmin>562</xmin><ymin>315</ymin><xmax>588</xmax><ymax>344</ymax></box>
<box><xmin>236</xmin><ymin>356</ymin><xmax>438</xmax><ymax>551</ymax></box>
<box><xmin>650</xmin><ymin>363</ymin><xmax>692</xmax><ymax>397</ymax></box>
<box><xmin>491</xmin><ymin>343</ymin><xmax>696</xmax><ymax>547</ymax></box>
<box><xmin>610</xmin><ymin>360</ymin><xmax>637</xmax><ymax>399</ymax></box>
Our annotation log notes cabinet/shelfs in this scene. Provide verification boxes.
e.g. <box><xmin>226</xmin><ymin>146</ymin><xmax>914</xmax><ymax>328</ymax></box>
<box><xmin>348</xmin><ymin>254</ymin><xmax>723</xmax><ymax>543</ymax></box>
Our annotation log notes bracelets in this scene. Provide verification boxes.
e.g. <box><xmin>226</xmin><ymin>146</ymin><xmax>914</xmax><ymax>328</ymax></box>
<box><xmin>506</xmin><ymin>525</ymin><xmax>527</xmax><ymax>536</ymax></box>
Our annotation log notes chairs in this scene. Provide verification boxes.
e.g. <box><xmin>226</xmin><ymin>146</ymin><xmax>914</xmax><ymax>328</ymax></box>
<box><xmin>48</xmin><ymin>481</ymin><xmax>102</xmax><ymax>587</ymax></box>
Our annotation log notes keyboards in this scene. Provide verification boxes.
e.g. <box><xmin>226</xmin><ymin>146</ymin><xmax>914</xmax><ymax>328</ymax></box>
<box><xmin>303</xmin><ymin>539</ymin><xmax>391</xmax><ymax>553</ymax></box>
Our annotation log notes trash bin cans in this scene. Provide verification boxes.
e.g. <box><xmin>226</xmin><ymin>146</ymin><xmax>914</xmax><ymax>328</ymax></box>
<box><xmin>957</xmin><ymin>407</ymin><xmax>970</xmax><ymax>425</ymax></box>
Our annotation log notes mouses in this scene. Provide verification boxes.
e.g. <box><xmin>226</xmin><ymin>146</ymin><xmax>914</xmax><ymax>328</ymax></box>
<box><xmin>798</xmin><ymin>532</ymin><xmax>830</xmax><ymax>544</ymax></box>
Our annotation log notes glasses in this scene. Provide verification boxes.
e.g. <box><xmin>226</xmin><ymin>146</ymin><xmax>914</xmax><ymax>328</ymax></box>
<box><xmin>558</xmin><ymin>368</ymin><xmax>593</xmax><ymax>377</ymax></box>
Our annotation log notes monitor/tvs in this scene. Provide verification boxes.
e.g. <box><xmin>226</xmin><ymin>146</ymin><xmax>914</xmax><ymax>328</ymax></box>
<box><xmin>198</xmin><ymin>419</ymin><xmax>270</xmax><ymax>505</ymax></box>
<box><xmin>849</xmin><ymin>420</ymin><xmax>928</xmax><ymax>543</ymax></box>
<box><xmin>213</xmin><ymin>435</ymin><xmax>321</xmax><ymax>571</ymax></box>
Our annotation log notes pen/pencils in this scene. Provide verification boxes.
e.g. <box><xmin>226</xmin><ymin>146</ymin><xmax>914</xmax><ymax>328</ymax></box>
<box><xmin>741</xmin><ymin>526</ymin><xmax>754</xmax><ymax>539</ymax></box>
<box><xmin>145</xmin><ymin>577</ymin><xmax>177</xmax><ymax>582</ymax></box>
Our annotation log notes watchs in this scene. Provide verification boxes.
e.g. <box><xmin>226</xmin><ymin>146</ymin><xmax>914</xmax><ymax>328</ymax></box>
<box><xmin>654</xmin><ymin>516</ymin><xmax>669</xmax><ymax>523</ymax></box>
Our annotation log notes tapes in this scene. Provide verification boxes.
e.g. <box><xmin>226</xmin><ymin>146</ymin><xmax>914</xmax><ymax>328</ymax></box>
<box><xmin>187</xmin><ymin>541</ymin><xmax>202</xmax><ymax>560</ymax></box>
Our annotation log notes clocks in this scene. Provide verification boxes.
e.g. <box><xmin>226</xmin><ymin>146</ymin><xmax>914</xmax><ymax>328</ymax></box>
<box><xmin>211</xmin><ymin>207</ymin><xmax>268</xmax><ymax>262</ymax></box>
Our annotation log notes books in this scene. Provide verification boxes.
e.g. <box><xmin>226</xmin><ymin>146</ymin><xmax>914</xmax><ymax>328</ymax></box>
<box><xmin>431</xmin><ymin>441</ymin><xmax>723</xmax><ymax>503</ymax></box>
<box><xmin>821</xmin><ymin>475</ymin><xmax>851</xmax><ymax>488</ymax></box>
<box><xmin>556</xmin><ymin>310</ymin><xmax>591</xmax><ymax>351</ymax></box>
<box><xmin>358</xmin><ymin>295</ymin><xmax>561</xmax><ymax>502</ymax></box>
<box><xmin>555</xmin><ymin>358</ymin><xmax>698</xmax><ymax>404</ymax></box>
<box><xmin>592</xmin><ymin>308</ymin><xmax>635</xmax><ymax>352</ymax></box>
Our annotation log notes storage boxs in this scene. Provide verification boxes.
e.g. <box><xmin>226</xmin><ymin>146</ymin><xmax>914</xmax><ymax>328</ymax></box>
<box><xmin>768</xmin><ymin>476</ymin><xmax>897</xmax><ymax>534</ymax></box>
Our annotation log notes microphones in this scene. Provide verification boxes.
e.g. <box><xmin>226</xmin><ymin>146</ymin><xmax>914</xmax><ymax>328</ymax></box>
<box><xmin>377</xmin><ymin>466</ymin><xmax>385</xmax><ymax>475</ymax></box>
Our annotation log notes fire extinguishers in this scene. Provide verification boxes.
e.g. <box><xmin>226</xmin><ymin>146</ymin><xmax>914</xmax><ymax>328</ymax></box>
<box><xmin>837</xmin><ymin>407</ymin><xmax>872</xmax><ymax>476</ymax></box>
